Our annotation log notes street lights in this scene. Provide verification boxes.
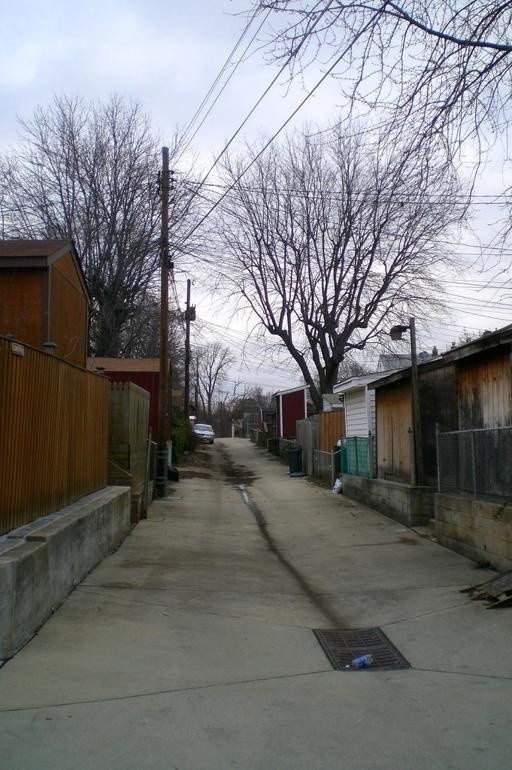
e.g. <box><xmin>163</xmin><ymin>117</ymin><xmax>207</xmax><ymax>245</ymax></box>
<box><xmin>387</xmin><ymin>314</ymin><xmax>428</xmax><ymax>485</ymax></box>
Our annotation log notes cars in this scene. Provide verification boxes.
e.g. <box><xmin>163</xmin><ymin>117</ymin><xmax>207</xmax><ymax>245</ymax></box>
<box><xmin>192</xmin><ymin>420</ymin><xmax>217</xmax><ymax>445</ymax></box>
